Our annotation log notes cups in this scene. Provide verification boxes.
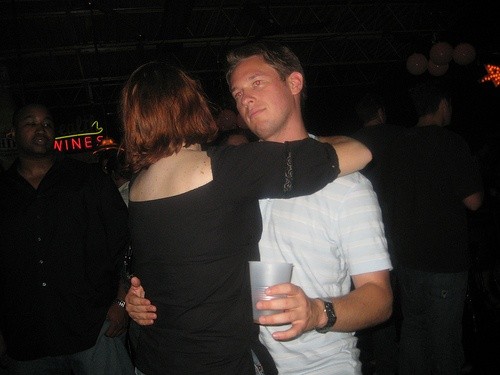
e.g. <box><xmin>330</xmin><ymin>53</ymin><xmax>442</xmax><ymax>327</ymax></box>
<box><xmin>249</xmin><ymin>261</ymin><xmax>293</xmax><ymax>322</ymax></box>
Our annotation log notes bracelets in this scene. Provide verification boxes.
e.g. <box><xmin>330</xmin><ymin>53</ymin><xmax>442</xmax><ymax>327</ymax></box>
<box><xmin>315</xmin><ymin>297</ymin><xmax>336</xmax><ymax>334</ymax></box>
<box><xmin>111</xmin><ymin>296</ymin><xmax>126</xmax><ymax>310</ymax></box>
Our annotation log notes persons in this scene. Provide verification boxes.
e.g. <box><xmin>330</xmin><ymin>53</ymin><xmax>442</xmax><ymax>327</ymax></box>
<box><xmin>218</xmin><ymin>82</ymin><xmax>486</xmax><ymax>375</ymax></box>
<box><xmin>118</xmin><ymin>60</ymin><xmax>373</xmax><ymax>375</ymax></box>
<box><xmin>0</xmin><ymin>96</ymin><xmax>133</xmax><ymax>375</ymax></box>
<box><xmin>124</xmin><ymin>42</ymin><xmax>395</xmax><ymax>375</ymax></box>
